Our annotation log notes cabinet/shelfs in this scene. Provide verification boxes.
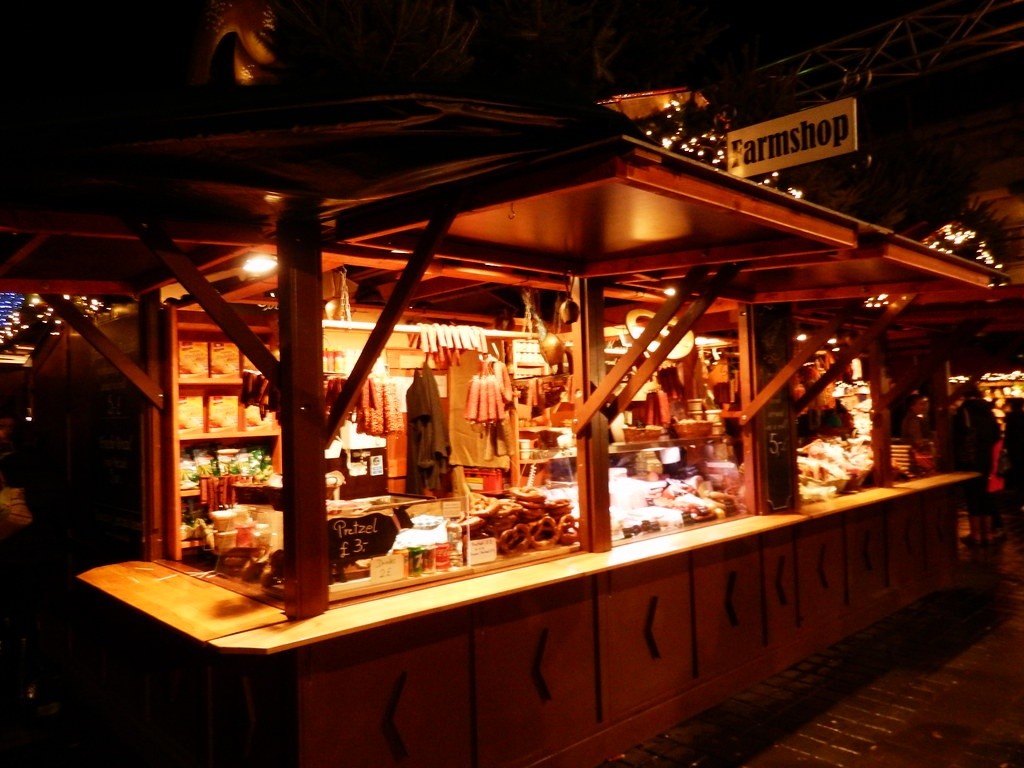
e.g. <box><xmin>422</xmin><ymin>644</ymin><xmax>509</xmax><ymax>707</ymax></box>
<box><xmin>163</xmin><ymin>300</ymin><xmax>282</xmax><ymax>561</ymax></box>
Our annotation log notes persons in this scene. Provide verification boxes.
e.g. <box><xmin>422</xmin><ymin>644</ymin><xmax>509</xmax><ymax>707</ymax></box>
<box><xmin>827</xmin><ymin>407</ymin><xmax>848</xmax><ymax>428</ymax></box>
<box><xmin>898</xmin><ymin>393</ymin><xmax>933</xmax><ymax>450</ymax></box>
<box><xmin>945</xmin><ymin>387</ymin><xmax>1001</xmax><ymax>547</ymax></box>
<box><xmin>1004</xmin><ymin>398</ymin><xmax>1024</xmax><ymax>511</ymax></box>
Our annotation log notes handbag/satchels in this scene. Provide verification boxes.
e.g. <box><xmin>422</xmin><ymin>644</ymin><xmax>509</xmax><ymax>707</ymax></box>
<box><xmin>988</xmin><ymin>440</ymin><xmax>1004</xmax><ymax>493</ymax></box>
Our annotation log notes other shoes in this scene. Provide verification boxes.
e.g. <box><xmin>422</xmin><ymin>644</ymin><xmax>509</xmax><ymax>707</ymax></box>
<box><xmin>960</xmin><ymin>530</ymin><xmax>1007</xmax><ymax>546</ymax></box>
